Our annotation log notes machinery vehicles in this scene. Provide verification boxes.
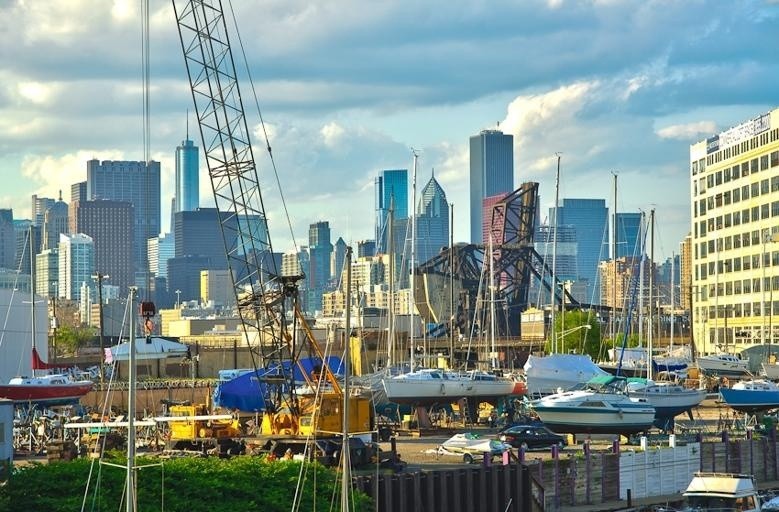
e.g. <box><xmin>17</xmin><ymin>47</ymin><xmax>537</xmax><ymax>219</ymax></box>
<box><xmin>167</xmin><ymin>405</ymin><xmax>244</xmax><ymax>455</ymax></box>
<box><xmin>170</xmin><ymin>3</ymin><xmax>382</xmax><ymax>462</ymax></box>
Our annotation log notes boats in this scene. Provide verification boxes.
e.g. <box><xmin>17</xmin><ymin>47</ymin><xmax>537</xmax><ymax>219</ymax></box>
<box><xmin>719</xmin><ymin>283</ymin><xmax>779</xmax><ymax>407</ymax></box>
<box><xmin>438</xmin><ymin>431</ymin><xmax>512</xmax><ymax>457</ymax></box>
<box><xmin>673</xmin><ymin>472</ymin><xmax>760</xmax><ymax>512</ymax></box>
<box><xmin>598</xmin><ymin>261</ymin><xmax>674</xmax><ymax>379</ymax></box>
<box><xmin>531</xmin><ymin>172</ymin><xmax>656</xmax><ymax>427</ymax></box>
<box><xmin>521</xmin><ymin>210</ymin><xmax>656</xmax><ymax>435</ymax></box>
<box><xmin>760</xmin><ymin>234</ymin><xmax>779</xmax><ymax>383</ymax></box>
<box><xmin>316</xmin><ymin>188</ymin><xmax>422</xmax><ymax>340</ymax></box>
<box><xmin>608</xmin><ymin>211</ymin><xmax>659</xmax><ymax>365</ymax></box>
<box><xmin>678</xmin><ymin>476</ymin><xmax>779</xmax><ymax>512</ymax></box>
<box><xmin>720</xmin><ymin>236</ymin><xmax>779</xmax><ymax>407</ymax></box>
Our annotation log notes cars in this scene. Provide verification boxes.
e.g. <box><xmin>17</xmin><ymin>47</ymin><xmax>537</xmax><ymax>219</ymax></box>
<box><xmin>499</xmin><ymin>424</ymin><xmax>567</xmax><ymax>454</ymax></box>
